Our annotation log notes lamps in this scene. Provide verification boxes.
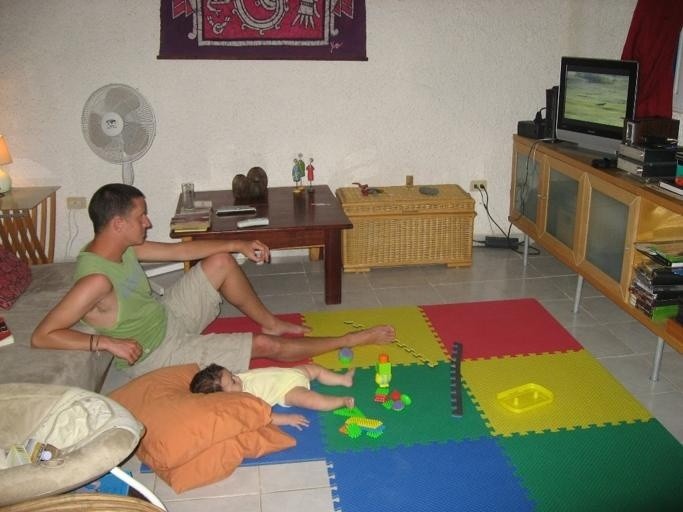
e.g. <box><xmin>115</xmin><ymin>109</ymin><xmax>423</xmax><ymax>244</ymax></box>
<box><xmin>0</xmin><ymin>132</ymin><xmax>13</xmax><ymax>194</ymax></box>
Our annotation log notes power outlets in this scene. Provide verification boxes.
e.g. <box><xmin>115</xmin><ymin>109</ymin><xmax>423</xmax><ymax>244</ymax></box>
<box><xmin>66</xmin><ymin>197</ymin><xmax>87</xmax><ymax>210</ymax></box>
<box><xmin>469</xmin><ymin>181</ymin><xmax>487</xmax><ymax>195</ymax></box>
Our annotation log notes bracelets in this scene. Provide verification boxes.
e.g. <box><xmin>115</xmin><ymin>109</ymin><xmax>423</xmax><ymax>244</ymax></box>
<box><xmin>89</xmin><ymin>334</ymin><xmax>99</xmax><ymax>353</ymax></box>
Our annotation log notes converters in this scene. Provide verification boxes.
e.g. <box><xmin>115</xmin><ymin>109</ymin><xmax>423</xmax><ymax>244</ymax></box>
<box><xmin>485</xmin><ymin>236</ymin><xmax>518</xmax><ymax>249</ymax></box>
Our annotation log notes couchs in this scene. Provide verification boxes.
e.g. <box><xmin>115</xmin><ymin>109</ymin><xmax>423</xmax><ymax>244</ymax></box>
<box><xmin>0</xmin><ymin>261</ymin><xmax>116</xmax><ymax>394</ymax></box>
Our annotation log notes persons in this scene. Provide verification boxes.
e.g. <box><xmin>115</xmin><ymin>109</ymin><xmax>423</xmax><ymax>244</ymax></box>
<box><xmin>190</xmin><ymin>361</ymin><xmax>356</xmax><ymax>431</ymax></box>
<box><xmin>29</xmin><ymin>183</ymin><xmax>398</xmax><ymax>377</ymax></box>
<box><xmin>291</xmin><ymin>153</ymin><xmax>315</xmax><ymax>188</ymax></box>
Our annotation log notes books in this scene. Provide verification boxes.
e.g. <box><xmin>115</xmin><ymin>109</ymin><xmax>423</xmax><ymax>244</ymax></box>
<box><xmin>0</xmin><ymin>314</ymin><xmax>15</xmax><ymax>346</ymax></box>
<box><xmin>628</xmin><ymin>240</ymin><xmax>682</xmax><ymax>323</ymax></box>
<box><xmin>169</xmin><ymin>205</ymin><xmax>214</xmax><ymax>233</ymax></box>
<box><xmin>659</xmin><ymin>175</ymin><xmax>683</xmax><ymax>196</ymax></box>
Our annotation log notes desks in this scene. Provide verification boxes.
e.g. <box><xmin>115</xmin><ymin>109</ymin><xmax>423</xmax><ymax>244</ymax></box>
<box><xmin>0</xmin><ymin>183</ymin><xmax>61</xmax><ymax>264</ymax></box>
<box><xmin>171</xmin><ymin>185</ymin><xmax>351</xmax><ymax>305</ymax></box>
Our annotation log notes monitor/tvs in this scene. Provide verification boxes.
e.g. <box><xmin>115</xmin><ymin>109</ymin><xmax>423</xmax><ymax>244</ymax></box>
<box><xmin>555</xmin><ymin>56</ymin><xmax>640</xmax><ymax>159</ymax></box>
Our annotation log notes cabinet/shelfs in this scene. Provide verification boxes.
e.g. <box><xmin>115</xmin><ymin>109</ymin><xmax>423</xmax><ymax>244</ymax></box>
<box><xmin>505</xmin><ymin>133</ymin><xmax>589</xmax><ymax>267</ymax></box>
<box><xmin>580</xmin><ymin>168</ymin><xmax>682</xmax><ymax>359</ymax></box>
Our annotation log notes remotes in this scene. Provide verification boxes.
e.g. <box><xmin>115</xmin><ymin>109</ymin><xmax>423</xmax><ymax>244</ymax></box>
<box><xmin>216</xmin><ymin>205</ymin><xmax>256</xmax><ymax>216</ymax></box>
<box><xmin>237</xmin><ymin>217</ymin><xmax>269</xmax><ymax>228</ymax></box>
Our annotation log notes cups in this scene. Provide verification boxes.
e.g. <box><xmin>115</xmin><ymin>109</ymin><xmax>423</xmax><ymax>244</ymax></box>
<box><xmin>182</xmin><ymin>184</ymin><xmax>194</xmax><ymax>209</ymax></box>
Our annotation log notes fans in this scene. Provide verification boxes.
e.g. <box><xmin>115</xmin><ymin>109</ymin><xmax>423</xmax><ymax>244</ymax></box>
<box><xmin>81</xmin><ymin>83</ymin><xmax>159</xmax><ymax>186</ymax></box>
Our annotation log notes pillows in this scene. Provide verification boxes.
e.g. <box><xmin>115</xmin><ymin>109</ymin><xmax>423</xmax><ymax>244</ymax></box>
<box><xmin>134</xmin><ymin>426</ymin><xmax>297</xmax><ymax>495</ymax></box>
<box><xmin>1</xmin><ymin>247</ymin><xmax>34</xmax><ymax>310</ymax></box>
<box><xmin>103</xmin><ymin>362</ymin><xmax>275</xmax><ymax>469</ymax></box>
